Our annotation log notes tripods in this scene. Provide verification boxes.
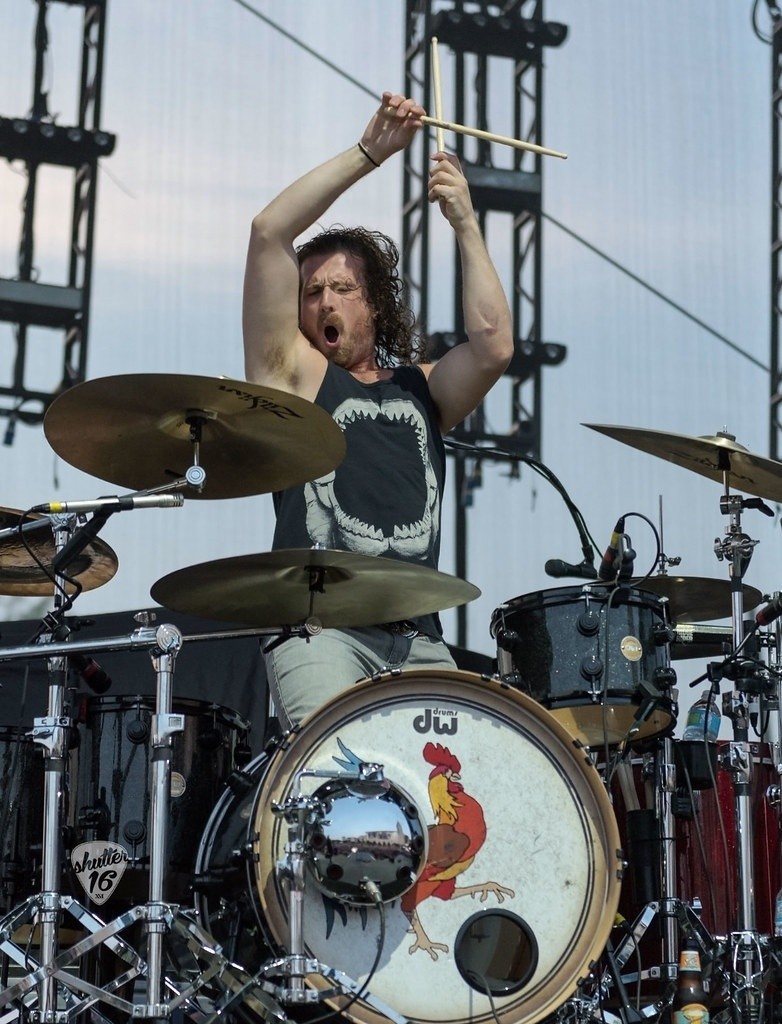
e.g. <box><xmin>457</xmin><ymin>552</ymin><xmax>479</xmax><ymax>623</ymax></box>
<box><xmin>0</xmin><ymin>431</ymin><xmax>413</xmax><ymax>1024</ymax></box>
<box><xmin>576</xmin><ymin>475</ymin><xmax>782</xmax><ymax>1024</ymax></box>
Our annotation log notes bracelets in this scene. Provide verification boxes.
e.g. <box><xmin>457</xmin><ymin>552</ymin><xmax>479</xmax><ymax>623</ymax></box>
<box><xmin>357</xmin><ymin>141</ymin><xmax>383</xmax><ymax>169</ymax></box>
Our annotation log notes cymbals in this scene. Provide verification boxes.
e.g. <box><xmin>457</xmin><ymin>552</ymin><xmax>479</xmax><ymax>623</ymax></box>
<box><xmin>0</xmin><ymin>505</ymin><xmax>120</xmax><ymax>597</ymax></box>
<box><xmin>578</xmin><ymin>422</ymin><xmax>781</xmax><ymax>505</ymax></box>
<box><xmin>149</xmin><ymin>548</ymin><xmax>482</xmax><ymax>628</ymax></box>
<box><xmin>626</xmin><ymin>575</ymin><xmax>763</xmax><ymax>623</ymax></box>
<box><xmin>42</xmin><ymin>372</ymin><xmax>348</xmax><ymax>501</ymax></box>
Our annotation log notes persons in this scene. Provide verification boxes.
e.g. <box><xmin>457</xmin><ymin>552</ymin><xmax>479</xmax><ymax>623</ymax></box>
<box><xmin>239</xmin><ymin>90</ymin><xmax>515</xmax><ymax>739</ymax></box>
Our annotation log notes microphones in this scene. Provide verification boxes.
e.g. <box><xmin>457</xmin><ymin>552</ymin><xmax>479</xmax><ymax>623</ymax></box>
<box><xmin>70</xmin><ymin>652</ymin><xmax>112</xmax><ymax>694</ymax></box>
<box><xmin>598</xmin><ymin>517</ymin><xmax>626</xmax><ymax>580</ymax></box>
<box><xmin>32</xmin><ymin>493</ymin><xmax>184</xmax><ymax>514</ymax></box>
<box><xmin>545</xmin><ymin>559</ymin><xmax>602</xmax><ymax>579</ymax></box>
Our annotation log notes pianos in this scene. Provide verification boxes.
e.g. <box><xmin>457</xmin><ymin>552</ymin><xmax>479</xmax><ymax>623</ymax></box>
<box><xmin>668</xmin><ymin>624</ymin><xmax>778</xmax><ymax>661</ymax></box>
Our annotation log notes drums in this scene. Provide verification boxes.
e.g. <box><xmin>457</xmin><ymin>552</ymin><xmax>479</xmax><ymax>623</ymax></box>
<box><xmin>488</xmin><ymin>579</ymin><xmax>679</xmax><ymax>748</ymax></box>
<box><xmin>59</xmin><ymin>692</ymin><xmax>255</xmax><ymax>934</ymax></box>
<box><xmin>0</xmin><ymin>722</ymin><xmax>81</xmax><ymax>928</ymax></box>
<box><xmin>191</xmin><ymin>665</ymin><xmax>628</xmax><ymax>1024</ymax></box>
<box><xmin>591</xmin><ymin>738</ymin><xmax>782</xmax><ymax>1024</ymax></box>
